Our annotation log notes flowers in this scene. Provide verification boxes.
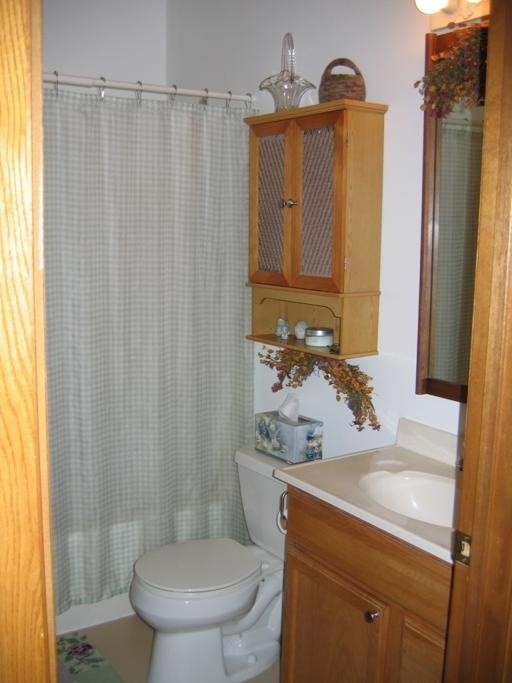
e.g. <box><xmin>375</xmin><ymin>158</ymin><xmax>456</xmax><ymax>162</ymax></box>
<box><xmin>258</xmin><ymin>348</ymin><xmax>383</xmax><ymax>433</ymax></box>
<box><xmin>416</xmin><ymin>25</ymin><xmax>484</xmax><ymax>120</ymax></box>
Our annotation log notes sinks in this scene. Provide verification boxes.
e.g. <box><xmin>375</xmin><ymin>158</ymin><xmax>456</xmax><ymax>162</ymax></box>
<box><xmin>360</xmin><ymin>469</ymin><xmax>456</xmax><ymax>526</ymax></box>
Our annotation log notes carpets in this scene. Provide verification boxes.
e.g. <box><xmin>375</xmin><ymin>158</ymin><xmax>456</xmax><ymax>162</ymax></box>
<box><xmin>56</xmin><ymin>630</ymin><xmax>122</xmax><ymax>683</ymax></box>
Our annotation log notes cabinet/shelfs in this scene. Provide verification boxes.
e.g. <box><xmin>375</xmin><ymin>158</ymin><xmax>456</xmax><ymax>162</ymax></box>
<box><xmin>243</xmin><ymin>99</ymin><xmax>387</xmax><ymax>362</ymax></box>
<box><xmin>281</xmin><ymin>489</ymin><xmax>450</xmax><ymax>682</ymax></box>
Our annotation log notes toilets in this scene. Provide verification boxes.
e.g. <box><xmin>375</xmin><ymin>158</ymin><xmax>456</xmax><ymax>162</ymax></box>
<box><xmin>129</xmin><ymin>443</ymin><xmax>290</xmax><ymax>683</ymax></box>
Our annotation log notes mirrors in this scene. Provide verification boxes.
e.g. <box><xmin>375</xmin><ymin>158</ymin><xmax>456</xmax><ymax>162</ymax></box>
<box><xmin>415</xmin><ymin>21</ymin><xmax>490</xmax><ymax>404</ymax></box>
